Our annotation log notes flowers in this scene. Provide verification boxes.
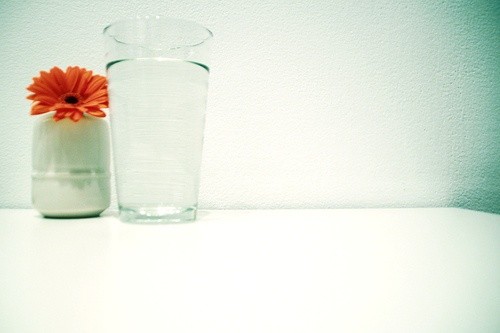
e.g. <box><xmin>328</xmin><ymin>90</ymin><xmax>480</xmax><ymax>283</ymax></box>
<box><xmin>27</xmin><ymin>66</ymin><xmax>109</xmax><ymax>122</ymax></box>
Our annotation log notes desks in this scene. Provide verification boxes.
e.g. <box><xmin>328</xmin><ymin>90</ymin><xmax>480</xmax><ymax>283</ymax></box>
<box><xmin>0</xmin><ymin>208</ymin><xmax>500</xmax><ymax>332</ymax></box>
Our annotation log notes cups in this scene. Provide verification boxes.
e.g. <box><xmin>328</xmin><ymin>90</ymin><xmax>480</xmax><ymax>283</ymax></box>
<box><xmin>102</xmin><ymin>15</ymin><xmax>215</xmax><ymax>225</ymax></box>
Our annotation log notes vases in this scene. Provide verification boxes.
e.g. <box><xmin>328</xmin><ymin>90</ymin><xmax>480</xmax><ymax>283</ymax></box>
<box><xmin>31</xmin><ymin>111</ymin><xmax>111</xmax><ymax>217</ymax></box>
